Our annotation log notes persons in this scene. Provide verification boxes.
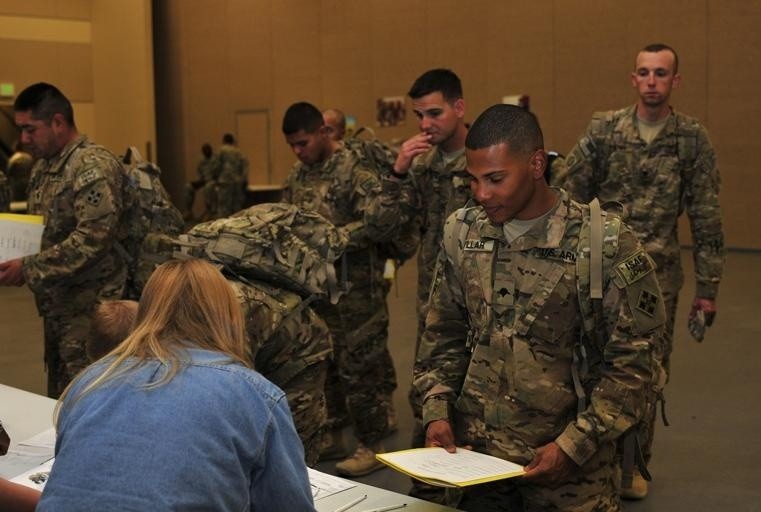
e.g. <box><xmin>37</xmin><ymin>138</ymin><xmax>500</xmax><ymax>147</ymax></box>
<box><xmin>548</xmin><ymin>43</ymin><xmax>725</xmax><ymax>498</ymax></box>
<box><xmin>0</xmin><ymin>477</ymin><xmax>44</xmax><ymax>512</ymax></box>
<box><xmin>391</xmin><ymin>68</ymin><xmax>478</xmax><ymax>497</ymax></box>
<box><xmin>96</xmin><ymin>101</ymin><xmax>398</xmax><ymax>479</ymax></box>
<box><xmin>405</xmin><ymin>103</ymin><xmax>668</xmax><ymax>511</ymax></box>
<box><xmin>35</xmin><ymin>256</ymin><xmax>318</xmax><ymax>511</ymax></box>
<box><xmin>0</xmin><ymin>81</ymin><xmax>185</xmax><ymax>402</ymax></box>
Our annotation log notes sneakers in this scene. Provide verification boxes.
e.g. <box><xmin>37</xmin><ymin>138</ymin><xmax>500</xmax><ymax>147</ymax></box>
<box><xmin>316</xmin><ymin>429</ymin><xmax>348</xmax><ymax>462</ymax></box>
<box><xmin>334</xmin><ymin>443</ymin><xmax>389</xmax><ymax>478</ymax></box>
<box><xmin>616</xmin><ymin>462</ymin><xmax>650</xmax><ymax>499</ymax></box>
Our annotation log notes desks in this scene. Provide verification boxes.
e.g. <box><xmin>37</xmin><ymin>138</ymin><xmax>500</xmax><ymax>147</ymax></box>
<box><xmin>1</xmin><ymin>384</ymin><xmax>462</xmax><ymax>511</ymax></box>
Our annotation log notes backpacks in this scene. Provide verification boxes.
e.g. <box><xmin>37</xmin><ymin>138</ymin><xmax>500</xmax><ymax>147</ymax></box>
<box><xmin>82</xmin><ymin>142</ymin><xmax>180</xmax><ymax>293</ymax></box>
<box><xmin>622</xmin><ymin>357</ymin><xmax>665</xmax><ymax>464</ymax></box>
<box><xmin>173</xmin><ymin>203</ymin><xmax>351</xmax><ymax>305</ymax></box>
<box><xmin>344</xmin><ymin>137</ymin><xmax>423</xmax><ymax>262</ymax></box>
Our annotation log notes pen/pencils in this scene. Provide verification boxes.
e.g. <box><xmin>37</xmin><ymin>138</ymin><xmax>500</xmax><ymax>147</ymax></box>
<box><xmin>333</xmin><ymin>494</ymin><xmax>407</xmax><ymax>512</ymax></box>
